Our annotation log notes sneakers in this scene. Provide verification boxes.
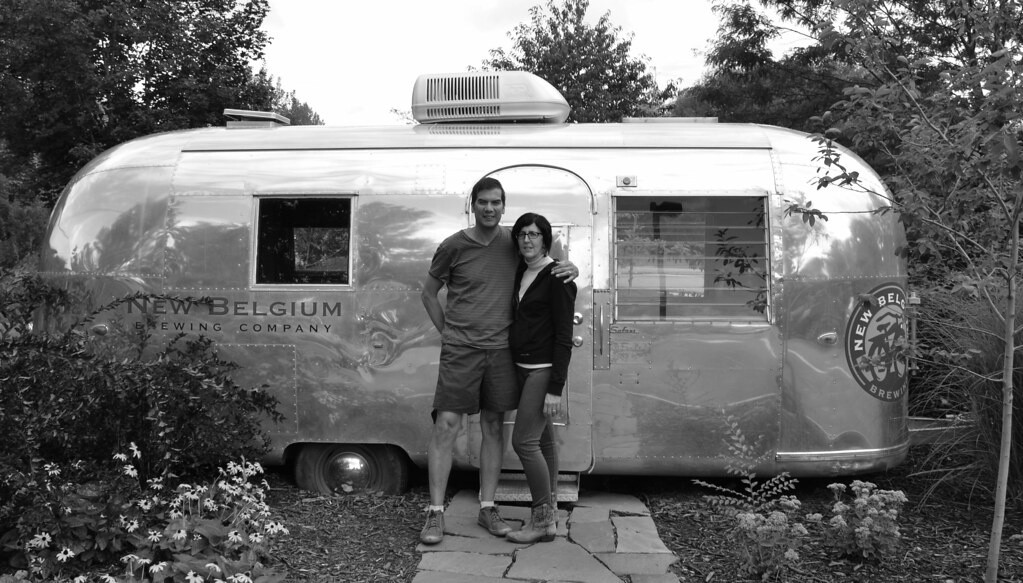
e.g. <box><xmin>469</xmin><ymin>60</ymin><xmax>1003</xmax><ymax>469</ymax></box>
<box><xmin>477</xmin><ymin>505</ymin><xmax>512</xmax><ymax>535</ymax></box>
<box><xmin>420</xmin><ymin>509</ymin><xmax>446</xmax><ymax>543</ymax></box>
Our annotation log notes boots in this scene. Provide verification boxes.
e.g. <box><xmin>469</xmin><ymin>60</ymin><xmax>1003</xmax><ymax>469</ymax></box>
<box><xmin>507</xmin><ymin>503</ymin><xmax>559</xmax><ymax>544</ymax></box>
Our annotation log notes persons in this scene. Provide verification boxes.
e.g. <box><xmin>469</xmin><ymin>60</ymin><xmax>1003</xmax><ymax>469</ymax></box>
<box><xmin>418</xmin><ymin>175</ymin><xmax>581</xmax><ymax>547</ymax></box>
<box><xmin>501</xmin><ymin>210</ymin><xmax>580</xmax><ymax>545</ymax></box>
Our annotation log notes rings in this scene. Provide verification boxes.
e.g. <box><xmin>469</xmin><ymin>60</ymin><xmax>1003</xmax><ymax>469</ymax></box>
<box><xmin>552</xmin><ymin>409</ymin><xmax>557</xmax><ymax>413</ymax></box>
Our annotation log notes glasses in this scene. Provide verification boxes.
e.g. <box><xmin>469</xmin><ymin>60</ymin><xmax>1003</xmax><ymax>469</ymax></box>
<box><xmin>516</xmin><ymin>231</ymin><xmax>541</xmax><ymax>239</ymax></box>
